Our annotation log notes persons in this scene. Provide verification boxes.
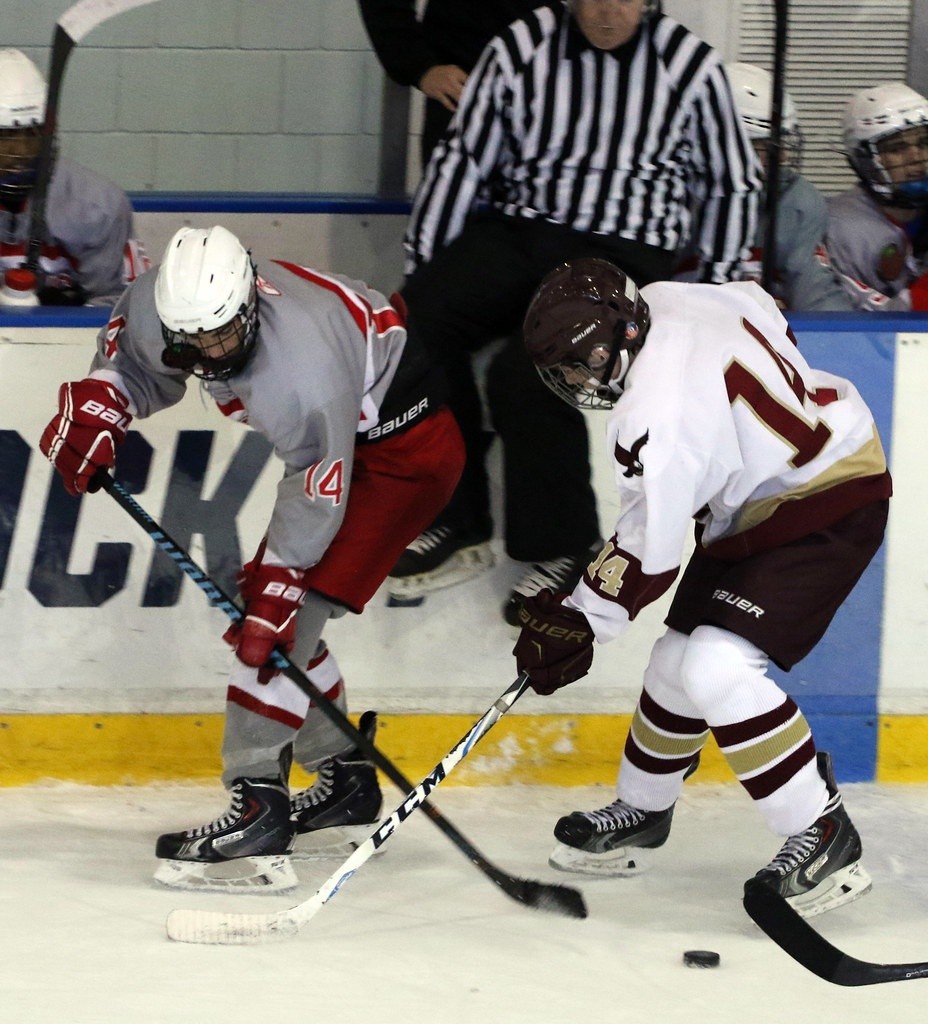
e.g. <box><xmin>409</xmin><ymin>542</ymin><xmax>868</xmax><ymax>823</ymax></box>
<box><xmin>0</xmin><ymin>46</ymin><xmax>156</xmax><ymax>308</ymax></box>
<box><xmin>717</xmin><ymin>59</ymin><xmax>854</xmax><ymax>313</ymax></box>
<box><xmin>354</xmin><ymin>0</ymin><xmax>547</xmax><ymax>178</ymax></box>
<box><xmin>36</xmin><ymin>224</ymin><xmax>467</xmax><ymax>899</ymax></box>
<box><xmin>384</xmin><ymin>0</ymin><xmax>764</xmax><ymax>629</ymax></box>
<box><xmin>508</xmin><ymin>257</ymin><xmax>895</xmax><ymax>918</ymax></box>
<box><xmin>825</xmin><ymin>79</ymin><xmax>928</xmax><ymax>313</ymax></box>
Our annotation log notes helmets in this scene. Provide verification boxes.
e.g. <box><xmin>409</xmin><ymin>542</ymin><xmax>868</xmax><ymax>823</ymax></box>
<box><xmin>843</xmin><ymin>82</ymin><xmax>927</xmax><ymax>211</ymax></box>
<box><xmin>520</xmin><ymin>260</ymin><xmax>648</xmax><ymax>409</ymax></box>
<box><xmin>156</xmin><ymin>225</ymin><xmax>259</xmax><ymax>380</ymax></box>
<box><xmin>727</xmin><ymin>60</ymin><xmax>800</xmax><ymax>201</ymax></box>
<box><xmin>2</xmin><ymin>49</ymin><xmax>51</xmax><ymax>207</ymax></box>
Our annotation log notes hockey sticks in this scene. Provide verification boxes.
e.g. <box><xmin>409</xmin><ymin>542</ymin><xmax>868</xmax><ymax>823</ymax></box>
<box><xmin>166</xmin><ymin>676</ymin><xmax>529</xmax><ymax>944</ymax></box>
<box><xmin>106</xmin><ymin>473</ymin><xmax>590</xmax><ymax>923</ymax></box>
<box><xmin>742</xmin><ymin>875</ymin><xmax>928</xmax><ymax>989</ymax></box>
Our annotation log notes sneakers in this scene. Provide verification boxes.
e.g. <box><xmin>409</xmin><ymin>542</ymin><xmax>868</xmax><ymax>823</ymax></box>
<box><xmin>505</xmin><ymin>540</ymin><xmax>604</xmax><ymax>639</ymax></box>
<box><xmin>287</xmin><ymin>712</ymin><xmax>380</xmax><ymax>858</ymax></box>
<box><xmin>745</xmin><ymin>751</ymin><xmax>871</xmax><ymax>919</ymax></box>
<box><xmin>153</xmin><ymin>744</ymin><xmax>299</xmax><ymax>895</ymax></box>
<box><xmin>549</xmin><ymin>755</ymin><xmax>701</xmax><ymax>873</ymax></box>
<box><xmin>386</xmin><ymin>511</ymin><xmax>499</xmax><ymax>602</ymax></box>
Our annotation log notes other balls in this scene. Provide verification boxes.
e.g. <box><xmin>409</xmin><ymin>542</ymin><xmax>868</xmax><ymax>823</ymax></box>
<box><xmin>683</xmin><ymin>949</ymin><xmax>722</xmax><ymax>971</ymax></box>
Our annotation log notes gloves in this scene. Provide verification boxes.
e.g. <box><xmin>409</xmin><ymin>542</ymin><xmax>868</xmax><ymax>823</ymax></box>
<box><xmin>227</xmin><ymin>559</ymin><xmax>308</xmax><ymax>684</ymax></box>
<box><xmin>38</xmin><ymin>379</ymin><xmax>132</xmax><ymax>495</ymax></box>
<box><xmin>508</xmin><ymin>603</ymin><xmax>593</xmax><ymax>697</ymax></box>
